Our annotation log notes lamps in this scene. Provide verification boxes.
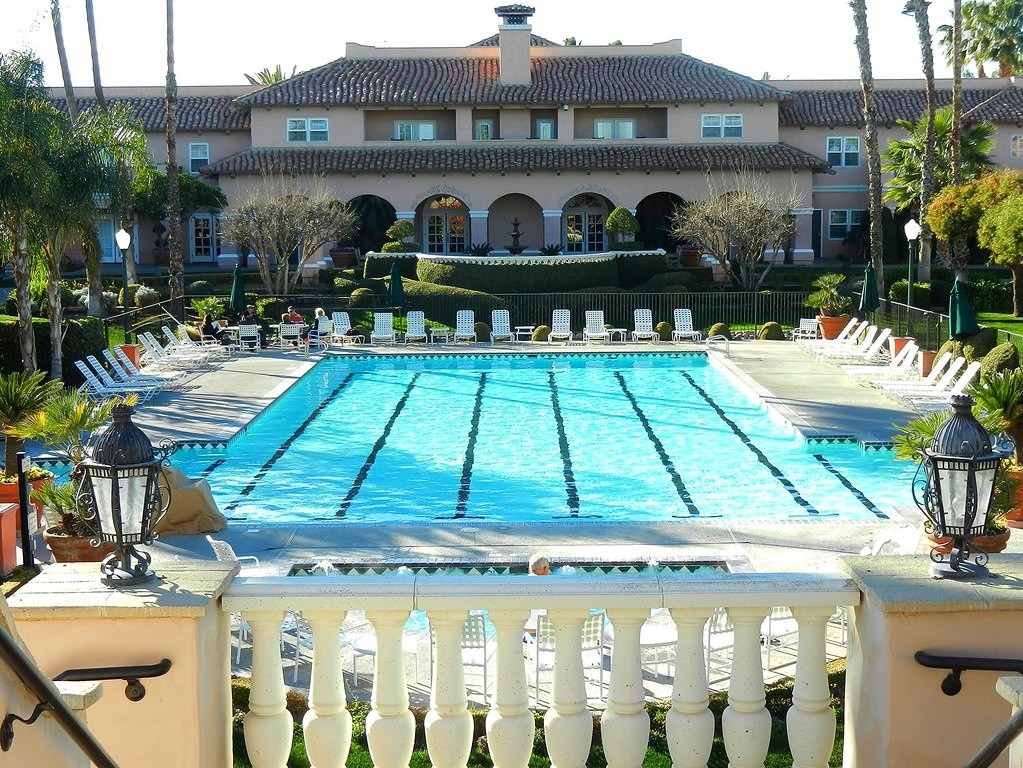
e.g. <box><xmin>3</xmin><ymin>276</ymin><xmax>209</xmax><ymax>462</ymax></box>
<box><xmin>73</xmin><ymin>403</ymin><xmax>172</xmax><ymax>585</ymax></box>
<box><xmin>563</xmin><ymin>103</ymin><xmax>568</xmax><ymax>111</ymax></box>
<box><xmin>907</xmin><ymin>394</ymin><xmax>1015</xmax><ymax>583</ymax></box>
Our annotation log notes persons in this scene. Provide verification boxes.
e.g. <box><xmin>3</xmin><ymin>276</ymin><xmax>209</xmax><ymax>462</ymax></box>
<box><xmin>240</xmin><ymin>306</ymin><xmax>269</xmax><ymax>352</ymax></box>
<box><xmin>280</xmin><ymin>307</ymin><xmax>303</xmax><ymax>349</ymax></box>
<box><xmin>202</xmin><ymin>315</ymin><xmax>233</xmax><ymax>351</ymax></box>
<box><xmin>300</xmin><ymin>308</ymin><xmax>328</xmax><ymax>349</ymax></box>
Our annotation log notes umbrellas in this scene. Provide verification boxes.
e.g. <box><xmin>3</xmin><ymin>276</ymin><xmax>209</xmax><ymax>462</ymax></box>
<box><xmin>230</xmin><ymin>264</ymin><xmax>245</xmax><ymax>324</ymax></box>
<box><xmin>387</xmin><ymin>263</ymin><xmax>404</xmax><ymax>341</ymax></box>
<box><xmin>859</xmin><ymin>261</ymin><xmax>880</xmax><ymax>337</ymax></box>
<box><xmin>948</xmin><ymin>278</ymin><xmax>976</xmax><ymax>362</ymax></box>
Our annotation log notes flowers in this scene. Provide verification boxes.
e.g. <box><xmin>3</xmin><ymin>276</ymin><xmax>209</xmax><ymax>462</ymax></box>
<box><xmin>331</xmin><ymin>246</ymin><xmax>356</xmax><ymax>251</ymax></box>
<box><xmin>677</xmin><ymin>242</ymin><xmax>704</xmax><ymax>250</ymax></box>
<box><xmin>0</xmin><ymin>466</ymin><xmax>49</xmax><ymax>484</ymax></box>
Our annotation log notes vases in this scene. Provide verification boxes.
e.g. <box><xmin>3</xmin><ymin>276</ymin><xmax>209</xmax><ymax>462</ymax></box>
<box><xmin>680</xmin><ymin>250</ymin><xmax>703</xmax><ymax>266</ymax></box>
<box><xmin>0</xmin><ymin>472</ymin><xmax>54</xmax><ymax>536</ymax></box>
<box><xmin>330</xmin><ymin>250</ymin><xmax>356</xmax><ymax>266</ymax></box>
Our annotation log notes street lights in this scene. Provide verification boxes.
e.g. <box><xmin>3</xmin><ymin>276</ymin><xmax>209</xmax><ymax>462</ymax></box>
<box><xmin>115</xmin><ymin>226</ymin><xmax>132</xmax><ymax>344</ymax></box>
<box><xmin>903</xmin><ymin>217</ymin><xmax>922</xmax><ymax>336</ymax></box>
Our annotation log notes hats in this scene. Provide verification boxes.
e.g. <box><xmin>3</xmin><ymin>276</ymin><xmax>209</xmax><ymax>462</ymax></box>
<box><xmin>288</xmin><ymin>306</ymin><xmax>295</xmax><ymax>313</ymax></box>
<box><xmin>247</xmin><ymin>304</ymin><xmax>255</xmax><ymax>309</ymax></box>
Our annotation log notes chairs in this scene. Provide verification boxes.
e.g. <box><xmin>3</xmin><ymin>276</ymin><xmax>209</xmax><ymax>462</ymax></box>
<box><xmin>793</xmin><ymin>317</ymin><xmax>1003</xmax><ymax>417</ymax></box>
<box><xmin>332</xmin><ymin>307</ymin><xmax>703</xmax><ymax>346</ymax></box>
<box><xmin>73</xmin><ymin>313</ymin><xmax>332</xmax><ymax>416</ymax></box>
<box><xmin>204</xmin><ymin>534</ymin><xmax>844</xmax><ymax>704</ymax></box>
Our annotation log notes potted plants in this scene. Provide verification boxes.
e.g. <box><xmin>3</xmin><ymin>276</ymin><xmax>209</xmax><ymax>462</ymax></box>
<box><xmin>927</xmin><ymin>458</ymin><xmax>1011</xmax><ymax>555</ymax></box>
<box><xmin>804</xmin><ymin>274</ymin><xmax>852</xmax><ymax>341</ymax></box>
<box><xmin>152</xmin><ymin>223</ymin><xmax>171</xmax><ymax>266</ymax></box>
<box><xmin>191</xmin><ymin>296</ymin><xmax>227</xmax><ymax>328</ymax></box>
<box><xmin>892</xmin><ymin>364</ymin><xmax>1022</xmax><ymax>529</ymax></box>
<box><xmin>3</xmin><ymin>387</ymin><xmax>141</xmax><ymax>561</ymax></box>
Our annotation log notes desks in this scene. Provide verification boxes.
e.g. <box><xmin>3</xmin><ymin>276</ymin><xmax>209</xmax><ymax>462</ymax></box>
<box><xmin>215</xmin><ymin>323</ymin><xmax>309</xmax><ymax>343</ymax></box>
<box><xmin>607</xmin><ymin>328</ymin><xmax>628</xmax><ymax>342</ymax></box>
<box><xmin>429</xmin><ymin>327</ymin><xmax>451</xmax><ymax>343</ymax></box>
<box><xmin>514</xmin><ymin>326</ymin><xmax>536</xmax><ymax>343</ymax></box>
